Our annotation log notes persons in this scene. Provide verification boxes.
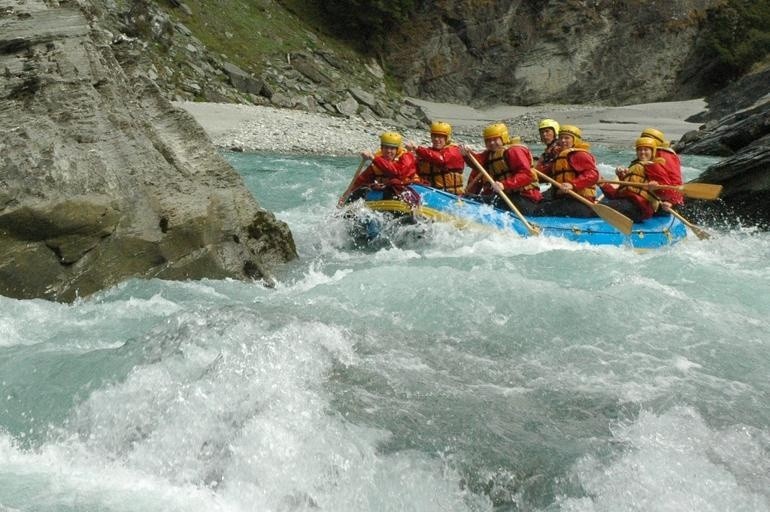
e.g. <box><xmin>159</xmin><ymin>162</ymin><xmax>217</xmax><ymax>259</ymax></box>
<box><xmin>640</xmin><ymin>127</ymin><xmax>687</xmax><ymax>217</ymax></box>
<box><xmin>335</xmin><ymin>132</ymin><xmax>417</xmax><ymax>207</ymax></box>
<box><xmin>462</xmin><ymin>118</ymin><xmax>599</xmax><ymax>219</ymax></box>
<box><xmin>592</xmin><ymin>137</ymin><xmax>672</xmax><ymax>224</ymax></box>
<box><xmin>404</xmin><ymin>121</ymin><xmax>465</xmax><ymax>197</ymax></box>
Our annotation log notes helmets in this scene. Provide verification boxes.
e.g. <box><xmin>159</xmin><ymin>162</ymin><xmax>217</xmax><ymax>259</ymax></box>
<box><xmin>559</xmin><ymin>125</ymin><xmax>582</xmax><ymax>146</ymax></box>
<box><xmin>381</xmin><ymin>132</ymin><xmax>401</xmax><ymax>155</ymax></box>
<box><xmin>642</xmin><ymin>128</ymin><xmax>664</xmax><ymax>147</ymax></box>
<box><xmin>539</xmin><ymin>119</ymin><xmax>560</xmax><ymax>140</ymax></box>
<box><xmin>636</xmin><ymin>137</ymin><xmax>657</xmax><ymax>161</ymax></box>
<box><xmin>431</xmin><ymin>122</ymin><xmax>451</xmax><ymax>143</ymax></box>
<box><xmin>484</xmin><ymin>123</ymin><xmax>509</xmax><ymax>145</ymax></box>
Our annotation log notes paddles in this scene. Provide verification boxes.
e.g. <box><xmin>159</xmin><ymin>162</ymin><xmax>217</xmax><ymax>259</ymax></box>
<box><xmin>533</xmin><ymin>169</ymin><xmax>634</xmax><ymax>234</ymax></box>
<box><xmin>660</xmin><ymin>201</ymin><xmax>715</xmax><ymax>241</ymax></box>
<box><xmin>599</xmin><ymin>180</ymin><xmax>722</xmax><ymax>199</ymax></box>
<box><xmin>466</xmin><ymin>152</ymin><xmax>540</xmax><ymax>236</ymax></box>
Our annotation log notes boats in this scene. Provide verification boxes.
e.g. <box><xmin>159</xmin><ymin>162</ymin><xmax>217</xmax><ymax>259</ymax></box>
<box><xmin>344</xmin><ymin>182</ymin><xmax>686</xmax><ymax>250</ymax></box>
